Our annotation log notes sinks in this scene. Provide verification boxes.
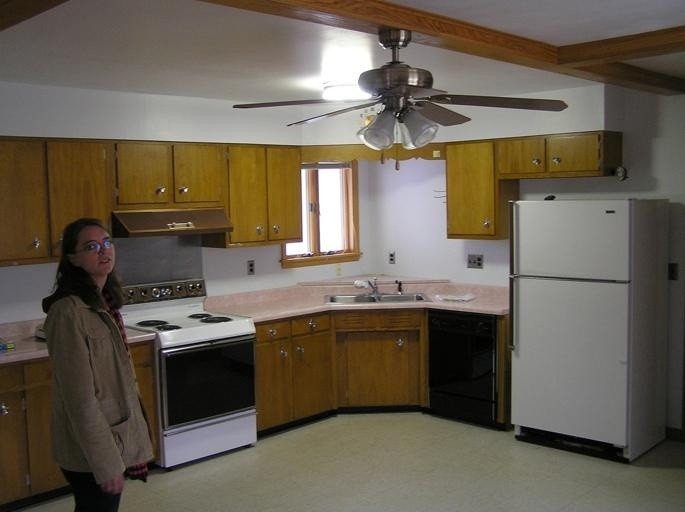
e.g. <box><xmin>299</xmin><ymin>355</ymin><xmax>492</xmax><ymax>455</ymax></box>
<box><xmin>378</xmin><ymin>294</ymin><xmax>434</xmax><ymax>303</ymax></box>
<box><xmin>323</xmin><ymin>293</ymin><xmax>378</xmax><ymax>303</ymax></box>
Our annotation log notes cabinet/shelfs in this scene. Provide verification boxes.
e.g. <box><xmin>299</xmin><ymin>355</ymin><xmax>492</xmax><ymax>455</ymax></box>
<box><xmin>25</xmin><ymin>340</ymin><xmax>161</xmax><ymax>498</ymax></box>
<box><xmin>496</xmin><ymin>130</ymin><xmax>622</xmax><ymax>180</ymax></box>
<box><xmin>1</xmin><ymin>137</ymin><xmax>115</xmax><ymax>266</ymax></box>
<box><xmin>445</xmin><ymin>140</ymin><xmax>519</xmax><ymax>239</ymax></box>
<box><xmin>116</xmin><ymin>141</ymin><xmax>228</xmax><ymax>209</ymax></box>
<box><xmin>228</xmin><ymin>143</ymin><xmax>302</xmax><ymax>246</ymax></box>
<box><xmin>0</xmin><ymin>362</ymin><xmax>32</xmax><ymax>508</ymax></box>
<box><xmin>335</xmin><ymin>308</ymin><xmax>430</xmax><ymax>409</ymax></box>
<box><xmin>255</xmin><ymin>309</ymin><xmax>335</xmax><ymax>434</ymax></box>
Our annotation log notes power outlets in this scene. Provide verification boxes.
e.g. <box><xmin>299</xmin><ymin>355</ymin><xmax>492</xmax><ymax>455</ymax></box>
<box><xmin>467</xmin><ymin>254</ymin><xmax>483</xmax><ymax>268</ymax></box>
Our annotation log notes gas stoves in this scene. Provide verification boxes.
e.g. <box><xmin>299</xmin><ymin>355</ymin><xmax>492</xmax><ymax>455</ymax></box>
<box><xmin>123</xmin><ymin>310</ymin><xmax>258</xmax><ymax>348</ymax></box>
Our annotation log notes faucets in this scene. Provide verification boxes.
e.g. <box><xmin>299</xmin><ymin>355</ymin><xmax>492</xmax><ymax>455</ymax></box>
<box><xmin>354</xmin><ymin>277</ymin><xmax>378</xmax><ymax>293</ymax></box>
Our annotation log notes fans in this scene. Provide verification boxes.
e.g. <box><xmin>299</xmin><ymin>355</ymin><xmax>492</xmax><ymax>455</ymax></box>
<box><xmin>233</xmin><ymin>30</ymin><xmax>568</xmax><ymax>127</ymax></box>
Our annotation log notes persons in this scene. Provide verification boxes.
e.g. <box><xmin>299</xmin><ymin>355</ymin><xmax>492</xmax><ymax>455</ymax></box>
<box><xmin>42</xmin><ymin>217</ymin><xmax>155</xmax><ymax>512</ymax></box>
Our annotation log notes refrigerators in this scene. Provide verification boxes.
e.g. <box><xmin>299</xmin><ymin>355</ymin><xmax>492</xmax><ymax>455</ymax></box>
<box><xmin>506</xmin><ymin>197</ymin><xmax>671</xmax><ymax>464</ymax></box>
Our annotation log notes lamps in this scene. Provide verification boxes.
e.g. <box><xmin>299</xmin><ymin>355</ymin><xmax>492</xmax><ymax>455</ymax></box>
<box><xmin>356</xmin><ymin>100</ymin><xmax>438</xmax><ymax>171</ymax></box>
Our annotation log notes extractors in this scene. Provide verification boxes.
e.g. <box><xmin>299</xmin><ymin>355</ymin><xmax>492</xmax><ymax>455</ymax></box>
<box><xmin>111</xmin><ymin>207</ymin><xmax>234</xmax><ymax>239</ymax></box>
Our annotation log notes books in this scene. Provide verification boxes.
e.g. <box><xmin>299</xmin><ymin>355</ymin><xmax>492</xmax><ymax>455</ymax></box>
<box><xmin>0</xmin><ymin>337</ymin><xmax>15</xmax><ymax>350</ymax></box>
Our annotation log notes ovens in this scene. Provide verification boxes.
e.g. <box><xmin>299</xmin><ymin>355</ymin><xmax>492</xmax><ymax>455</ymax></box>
<box><xmin>152</xmin><ymin>334</ymin><xmax>258</xmax><ymax>469</ymax></box>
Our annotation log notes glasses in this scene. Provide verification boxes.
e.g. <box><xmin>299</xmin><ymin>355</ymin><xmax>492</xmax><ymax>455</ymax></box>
<box><xmin>74</xmin><ymin>239</ymin><xmax>114</xmax><ymax>253</ymax></box>
